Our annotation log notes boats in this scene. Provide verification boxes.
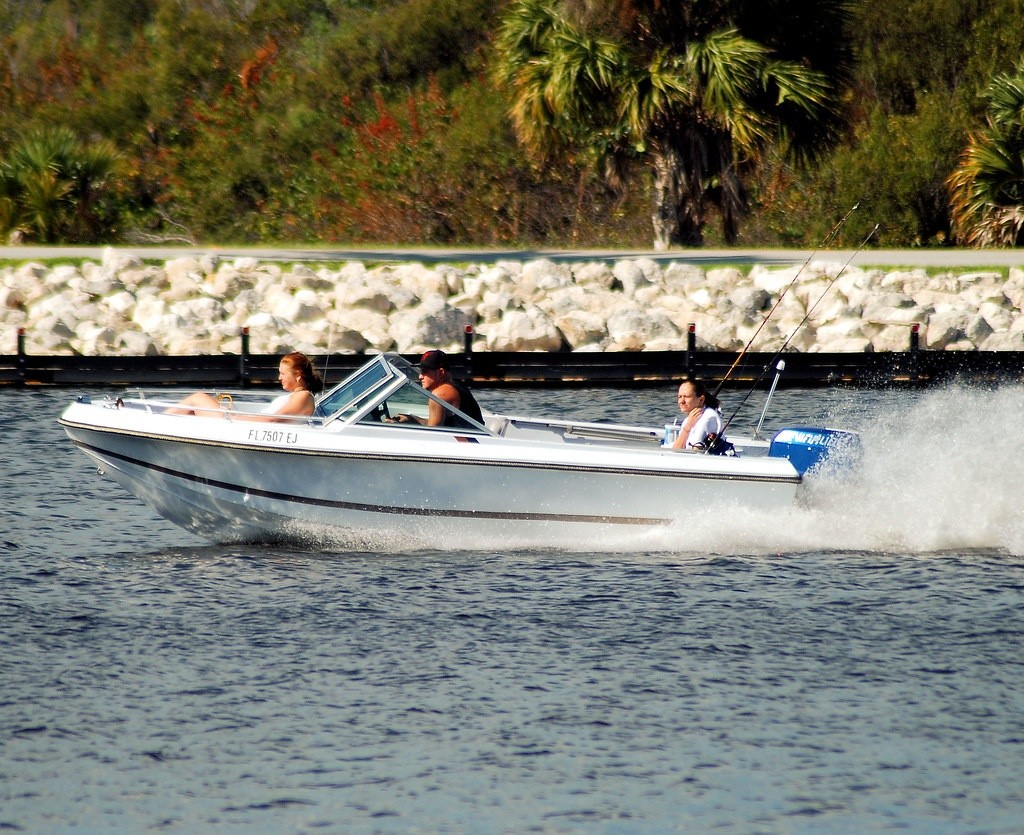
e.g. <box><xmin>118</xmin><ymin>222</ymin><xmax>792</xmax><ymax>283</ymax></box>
<box><xmin>56</xmin><ymin>198</ymin><xmax>884</xmax><ymax>556</ymax></box>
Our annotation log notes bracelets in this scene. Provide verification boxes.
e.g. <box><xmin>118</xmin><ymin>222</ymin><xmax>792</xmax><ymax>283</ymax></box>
<box><xmin>683</xmin><ymin>427</ymin><xmax>690</xmax><ymax>432</ymax></box>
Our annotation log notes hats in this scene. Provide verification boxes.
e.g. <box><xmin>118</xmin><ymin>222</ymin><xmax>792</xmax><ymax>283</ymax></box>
<box><xmin>410</xmin><ymin>351</ymin><xmax>448</xmax><ymax>370</ymax></box>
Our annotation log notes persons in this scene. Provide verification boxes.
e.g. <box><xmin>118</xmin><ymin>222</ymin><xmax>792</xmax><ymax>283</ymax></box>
<box><xmin>385</xmin><ymin>349</ymin><xmax>486</xmax><ymax>430</ymax></box>
<box><xmin>161</xmin><ymin>352</ymin><xmax>324</xmax><ymax>424</ymax></box>
<box><xmin>671</xmin><ymin>378</ymin><xmax>725</xmax><ymax>450</ymax></box>
